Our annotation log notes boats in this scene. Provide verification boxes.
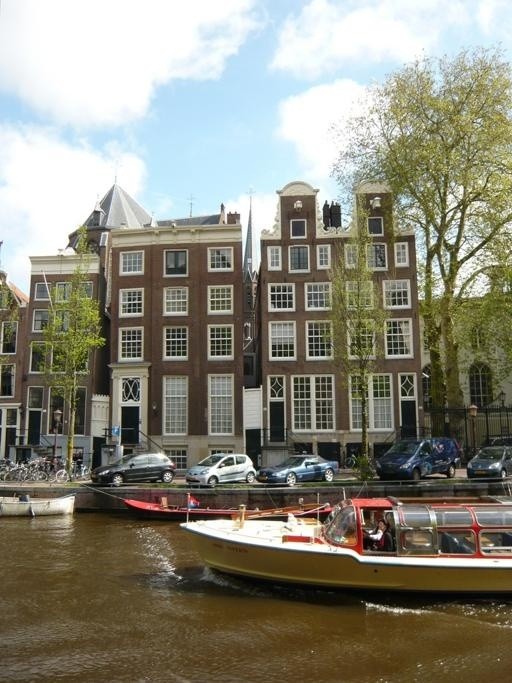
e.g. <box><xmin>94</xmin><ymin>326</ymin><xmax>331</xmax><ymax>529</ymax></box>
<box><xmin>122</xmin><ymin>498</ymin><xmax>342</xmax><ymax>521</ymax></box>
<box><xmin>0</xmin><ymin>492</ymin><xmax>76</xmax><ymax>516</ymax></box>
<box><xmin>176</xmin><ymin>492</ymin><xmax>512</xmax><ymax>598</ymax></box>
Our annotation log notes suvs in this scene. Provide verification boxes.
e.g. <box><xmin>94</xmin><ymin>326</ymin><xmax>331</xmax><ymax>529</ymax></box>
<box><xmin>468</xmin><ymin>436</ymin><xmax>511</xmax><ymax>459</ymax></box>
<box><xmin>90</xmin><ymin>451</ymin><xmax>176</xmax><ymax>486</ymax></box>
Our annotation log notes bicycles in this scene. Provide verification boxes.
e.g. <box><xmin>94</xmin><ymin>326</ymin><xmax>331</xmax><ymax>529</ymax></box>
<box><xmin>343</xmin><ymin>447</ymin><xmax>371</xmax><ymax>469</ymax></box>
<box><xmin>1</xmin><ymin>455</ymin><xmax>92</xmax><ymax>483</ymax></box>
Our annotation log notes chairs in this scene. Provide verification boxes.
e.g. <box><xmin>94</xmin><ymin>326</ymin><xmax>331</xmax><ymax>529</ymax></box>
<box><xmin>381</xmin><ymin>530</ymin><xmax>394</xmax><ymax>552</ymax></box>
<box><xmin>440</xmin><ymin>530</ymin><xmax>511</xmax><ymax>555</ymax></box>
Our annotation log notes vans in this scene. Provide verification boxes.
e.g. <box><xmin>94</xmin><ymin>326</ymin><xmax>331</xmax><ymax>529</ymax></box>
<box><xmin>466</xmin><ymin>444</ymin><xmax>512</xmax><ymax>481</ymax></box>
<box><xmin>374</xmin><ymin>436</ymin><xmax>461</xmax><ymax>483</ymax></box>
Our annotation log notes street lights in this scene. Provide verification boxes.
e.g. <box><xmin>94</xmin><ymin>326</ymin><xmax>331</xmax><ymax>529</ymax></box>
<box><xmin>498</xmin><ymin>389</ymin><xmax>506</xmax><ymax>407</ymax></box>
<box><xmin>52</xmin><ymin>408</ymin><xmax>63</xmax><ymax>466</ymax></box>
<box><xmin>469</xmin><ymin>403</ymin><xmax>478</xmax><ymax>452</ymax></box>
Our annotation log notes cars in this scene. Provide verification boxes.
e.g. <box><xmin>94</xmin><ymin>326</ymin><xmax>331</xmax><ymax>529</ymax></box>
<box><xmin>255</xmin><ymin>452</ymin><xmax>339</xmax><ymax>486</ymax></box>
<box><xmin>185</xmin><ymin>452</ymin><xmax>257</xmax><ymax>487</ymax></box>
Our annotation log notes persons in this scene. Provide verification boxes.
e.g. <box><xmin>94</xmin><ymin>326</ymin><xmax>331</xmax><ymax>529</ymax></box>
<box><xmin>362</xmin><ymin>519</ymin><xmax>383</xmax><ymax>551</ymax></box>
<box><xmin>372</xmin><ymin>517</ymin><xmax>395</xmax><ymax>552</ymax></box>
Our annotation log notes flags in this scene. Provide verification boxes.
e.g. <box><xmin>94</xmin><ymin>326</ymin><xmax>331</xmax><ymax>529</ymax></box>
<box><xmin>186</xmin><ymin>495</ymin><xmax>201</xmax><ymax>509</ymax></box>
